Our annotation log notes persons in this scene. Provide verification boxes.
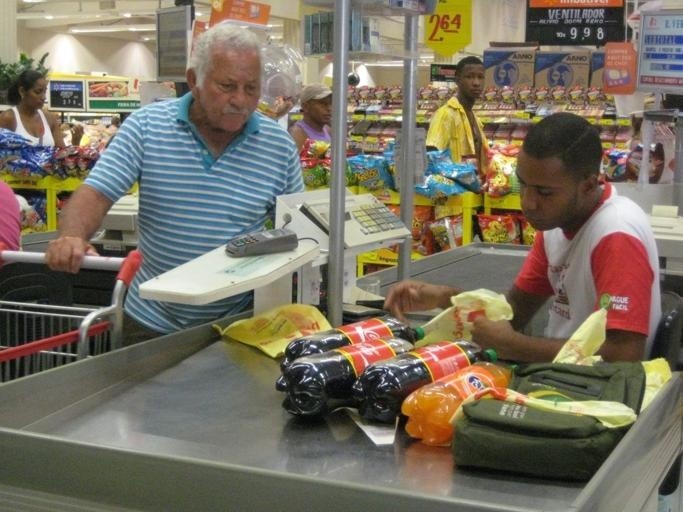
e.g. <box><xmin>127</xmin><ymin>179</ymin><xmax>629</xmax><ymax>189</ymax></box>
<box><xmin>0</xmin><ymin>174</ymin><xmax>22</xmax><ymax>267</ymax></box>
<box><xmin>425</xmin><ymin>56</ymin><xmax>493</xmax><ymax>183</ymax></box>
<box><xmin>384</xmin><ymin>112</ymin><xmax>663</xmax><ymax>365</ymax></box>
<box><xmin>0</xmin><ymin>70</ymin><xmax>84</xmax><ymax>149</ymax></box>
<box><xmin>287</xmin><ymin>82</ymin><xmax>332</xmax><ymax>154</ymax></box>
<box><xmin>44</xmin><ymin>23</ymin><xmax>306</xmax><ymax>349</ymax></box>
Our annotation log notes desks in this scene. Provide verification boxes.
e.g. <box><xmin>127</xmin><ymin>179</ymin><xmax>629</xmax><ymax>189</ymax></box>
<box><xmin>0</xmin><ymin>244</ymin><xmax>682</xmax><ymax>512</ymax></box>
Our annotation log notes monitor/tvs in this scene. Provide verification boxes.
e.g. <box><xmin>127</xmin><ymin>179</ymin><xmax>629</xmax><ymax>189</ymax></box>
<box><xmin>155</xmin><ymin>6</ymin><xmax>193</xmax><ymax>83</ymax></box>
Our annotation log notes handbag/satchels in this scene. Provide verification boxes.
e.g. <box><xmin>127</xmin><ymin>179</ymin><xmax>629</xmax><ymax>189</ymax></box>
<box><xmin>453</xmin><ymin>361</ymin><xmax>645</xmax><ymax>483</ymax></box>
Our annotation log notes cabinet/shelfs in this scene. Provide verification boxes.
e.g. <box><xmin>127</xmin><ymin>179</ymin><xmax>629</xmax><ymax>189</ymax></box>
<box><xmin>0</xmin><ymin>175</ymin><xmax>132</xmax><ymax>234</ymax></box>
<box><xmin>481</xmin><ymin>192</ymin><xmax>523</xmax><ymax>216</ymax></box>
<box><xmin>355</xmin><ymin>187</ymin><xmax>483</xmax><ymax>280</ymax></box>
<box><xmin>287</xmin><ymin>92</ymin><xmax>660</xmax><ymax>156</ymax></box>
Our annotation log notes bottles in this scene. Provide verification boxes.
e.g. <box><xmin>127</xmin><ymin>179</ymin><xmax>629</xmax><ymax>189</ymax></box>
<box><xmin>280</xmin><ymin>314</ymin><xmax>425</xmax><ymax>380</ymax></box>
<box><xmin>401</xmin><ymin>362</ymin><xmax>519</xmax><ymax>446</ymax></box>
<box><xmin>276</xmin><ymin>337</ymin><xmax>417</xmax><ymax>416</ymax></box>
<box><xmin>352</xmin><ymin>340</ymin><xmax>499</xmax><ymax>428</ymax></box>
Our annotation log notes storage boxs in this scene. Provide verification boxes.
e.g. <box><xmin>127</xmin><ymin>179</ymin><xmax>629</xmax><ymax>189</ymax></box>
<box><xmin>537</xmin><ymin>50</ymin><xmax>591</xmax><ymax>87</ymax></box>
<box><xmin>593</xmin><ymin>51</ymin><xmax>606</xmax><ymax>90</ymax></box>
<box><xmin>484</xmin><ymin>42</ymin><xmax>538</xmax><ymax>87</ymax></box>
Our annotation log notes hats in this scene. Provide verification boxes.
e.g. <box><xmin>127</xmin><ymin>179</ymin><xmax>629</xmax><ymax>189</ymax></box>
<box><xmin>301</xmin><ymin>84</ymin><xmax>332</xmax><ymax>103</ymax></box>
